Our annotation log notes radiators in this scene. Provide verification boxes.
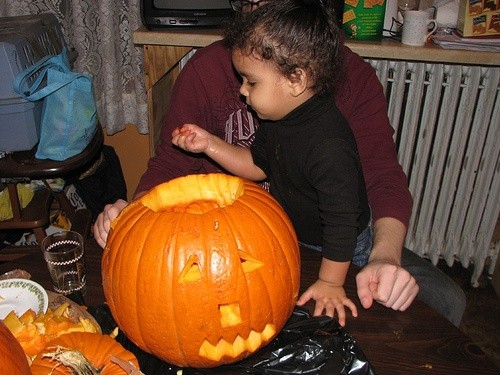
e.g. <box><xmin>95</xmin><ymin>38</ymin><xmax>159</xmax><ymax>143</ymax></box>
<box><xmin>364</xmin><ymin>58</ymin><xmax>500</xmax><ymax>289</ymax></box>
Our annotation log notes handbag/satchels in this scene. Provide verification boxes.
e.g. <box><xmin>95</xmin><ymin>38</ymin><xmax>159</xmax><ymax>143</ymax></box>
<box><xmin>12</xmin><ymin>54</ymin><xmax>98</xmax><ymax>161</ymax></box>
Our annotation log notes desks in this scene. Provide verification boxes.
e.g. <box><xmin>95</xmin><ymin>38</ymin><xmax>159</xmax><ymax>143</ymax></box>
<box><xmin>131</xmin><ymin>21</ymin><xmax>500</xmax><ymax>157</ymax></box>
<box><xmin>0</xmin><ymin>236</ymin><xmax>500</xmax><ymax>375</ymax></box>
<box><xmin>0</xmin><ymin>124</ymin><xmax>105</xmax><ymax>264</ymax></box>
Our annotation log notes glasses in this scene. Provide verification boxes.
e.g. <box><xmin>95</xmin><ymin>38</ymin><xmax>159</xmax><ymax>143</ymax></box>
<box><xmin>229</xmin><ymin>0</ymin><xmax>269</xmax><ymax>15</ymax></box>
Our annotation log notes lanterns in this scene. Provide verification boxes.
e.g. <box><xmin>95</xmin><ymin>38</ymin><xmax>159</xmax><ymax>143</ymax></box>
<box><xmin>101</xmin><ymin>173</ymin><xmax>301</xmax><ymax>368</ymax></box>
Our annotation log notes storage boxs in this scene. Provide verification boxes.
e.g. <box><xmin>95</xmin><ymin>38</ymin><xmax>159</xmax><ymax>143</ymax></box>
<box><xmin>343</xmin><ymin>0</ymin><xmax>388</xmax><ymax>43</ymax></box>
<box><xmin>0</xmin><ymin>13</ymin><xmax>78</xmax><ymax>152</ymax></box>
<box><xmin>457</xmin><ymin>0</ymin><xmax>500</xmax><ymax>38</ymax></box>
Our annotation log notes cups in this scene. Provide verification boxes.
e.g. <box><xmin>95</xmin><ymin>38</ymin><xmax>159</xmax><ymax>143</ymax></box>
<box><xmin>41</xmin><ymin>231</ymin><xmax>87</xmax><ymax>295</ymax></box>
<box><xmin>398</xmin><ymin>0</ymin><xmax>419</xmax><ymax>16</ymax></box>
<box><xmin>400</xmin><ymin>9</ymin><xmax>438</xmax><ymax>46</ymax></box>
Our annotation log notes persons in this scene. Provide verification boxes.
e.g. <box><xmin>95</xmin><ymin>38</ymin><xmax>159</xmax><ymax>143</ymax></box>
<box><xmin>171</xmin><ymin>0</ymin><xmax>358</xmax><ymax>327</ymax></box>
<box><xmin>94</xmin><ymin>0</ymin><xmax>420</xmax><ymax>312</ymax></box>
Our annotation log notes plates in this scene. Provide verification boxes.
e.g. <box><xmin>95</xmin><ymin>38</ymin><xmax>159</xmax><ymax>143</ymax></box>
<box><xmin>0</xmin><ymin>277</ymin><xmax>48</xmax><ymax>324</ymax></box>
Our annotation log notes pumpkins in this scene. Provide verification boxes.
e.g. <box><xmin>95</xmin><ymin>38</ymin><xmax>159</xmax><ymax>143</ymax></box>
<box><xmin>28</xmin><ymin>332</ymin><xmax>140</xmax><ymax>375</ymax></box>
<box><xmin>0</xmin><ymin>319</ymin><xmax>34</xmax><ymax>375</ymax></box>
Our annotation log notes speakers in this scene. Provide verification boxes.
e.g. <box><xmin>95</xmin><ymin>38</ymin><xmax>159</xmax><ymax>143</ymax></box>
<box><xmin>140</xmin><ymin>0</ymin><xmax>242</xmax><ymax>31</ymax></box>
